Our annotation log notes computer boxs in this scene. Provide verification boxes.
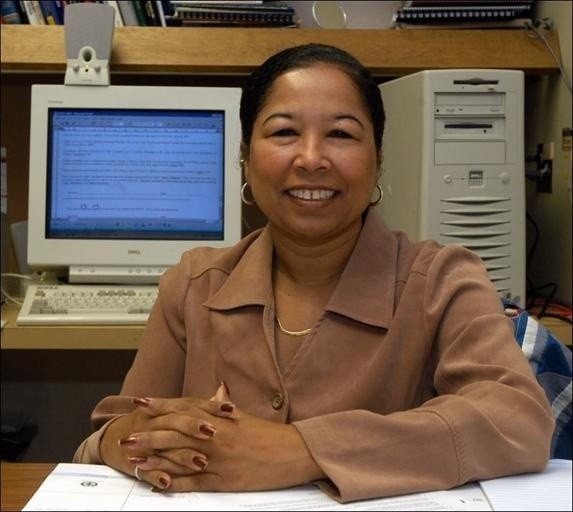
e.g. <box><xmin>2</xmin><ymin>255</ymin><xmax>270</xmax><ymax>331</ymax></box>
<box><xmin>370</xmin><ymin>68</ymin><xmax>526</xmax><ymax>310</ymax></box>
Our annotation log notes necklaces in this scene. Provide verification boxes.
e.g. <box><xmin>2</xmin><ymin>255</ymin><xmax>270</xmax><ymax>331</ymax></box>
<box><xmin>275</xmin><ymin>312</ymin><xmax>313</xmax><ymax>338</ymax></box>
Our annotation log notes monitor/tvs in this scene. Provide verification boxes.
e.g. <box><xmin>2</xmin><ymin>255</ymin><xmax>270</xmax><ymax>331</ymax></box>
<box><xmin>26</xmin><ymin>84</ymin><xmax>243</xmax><ymax>283</ymax></box>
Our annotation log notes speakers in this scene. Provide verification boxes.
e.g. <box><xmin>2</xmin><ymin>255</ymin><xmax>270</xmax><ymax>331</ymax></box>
<box><xmin>9</xmin><ymin>220</ymin><xmax>58</xmax><ymax>297</ymax></box>
<box><xmin>64</xmin><ymin>2</ymin><xmax>115</xmax><ymax>86</ymax></box>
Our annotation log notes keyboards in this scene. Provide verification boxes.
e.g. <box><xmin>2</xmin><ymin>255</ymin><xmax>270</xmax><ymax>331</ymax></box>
<box><xmin>16</xmin><ymin>284</ymin><xmax>160</xmax><ymax>326</ymax></box>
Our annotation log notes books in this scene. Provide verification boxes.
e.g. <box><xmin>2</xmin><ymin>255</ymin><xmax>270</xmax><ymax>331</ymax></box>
<box><xmin>0</xmin><ymin>0</ymin><xmax>299</xmax><ymax>29</ymax></box>
<box><xmin>393</xmin><ymin>0</ymin><xmax>537</xmax><ymax>28</ymax></box>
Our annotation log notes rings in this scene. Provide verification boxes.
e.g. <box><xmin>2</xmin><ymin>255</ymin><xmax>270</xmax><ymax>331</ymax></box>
<box><xmin>134</xmin><ymin>465</ymin><xmax>142</xmax><ymax>481</ymax></box>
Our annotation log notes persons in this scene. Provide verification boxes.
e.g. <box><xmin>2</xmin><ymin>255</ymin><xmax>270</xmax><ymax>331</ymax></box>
<box><xmin>71</xmin><ymin>42</ymin><xmax>555</xmax><ymax>494</ymax></box>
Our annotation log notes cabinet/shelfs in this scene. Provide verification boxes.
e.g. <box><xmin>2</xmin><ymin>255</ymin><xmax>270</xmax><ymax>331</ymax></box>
<box><xmin>1</xmin><ymin>18</ymin><xmax>572</xmax><ymax>360</ymax></box>
